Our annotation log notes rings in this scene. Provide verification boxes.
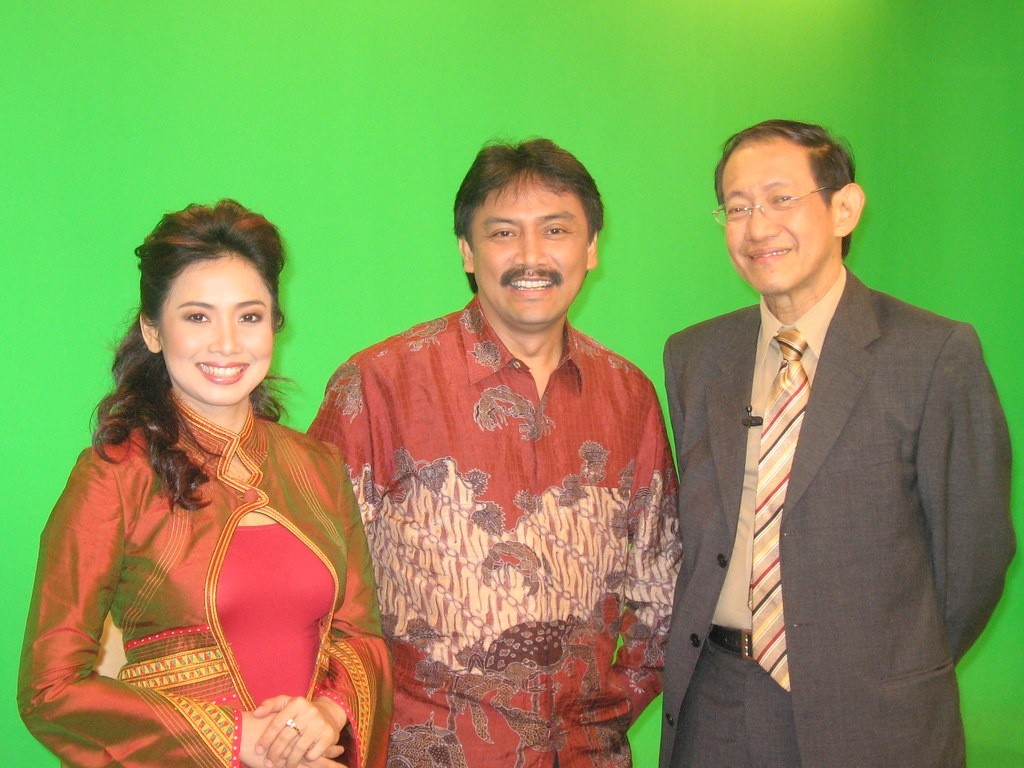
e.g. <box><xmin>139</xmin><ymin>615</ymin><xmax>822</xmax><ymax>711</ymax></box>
<box><xmin>286</xmin><ymin>719</ymin><xmax>301</xmax><ymax>736</ymax></box>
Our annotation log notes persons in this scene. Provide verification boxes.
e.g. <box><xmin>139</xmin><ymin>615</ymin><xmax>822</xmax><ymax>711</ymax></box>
<box><xmin>663</xmin><ymin>120</ymin><xmax>1016</xmax><ymax>766</ymax></box>
<box><xmin>308</xmin><ymin>137</ymin><xmax>680</xmax><ymax>767</ymax></box>
<box><xmin>15</xmin><ymin>198</ymin><xmax>392</xmax><ymax>768</ymax></box>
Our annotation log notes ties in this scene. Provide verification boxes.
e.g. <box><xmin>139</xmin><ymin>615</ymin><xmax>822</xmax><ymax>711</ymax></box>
<box><xmin>751</xmin><ymin>330</ymin><xmax>811</xmax><ymax>691</ymax></box>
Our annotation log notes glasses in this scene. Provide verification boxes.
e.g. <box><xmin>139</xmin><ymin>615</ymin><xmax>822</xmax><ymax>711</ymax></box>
<box><xmin>711</xmin><ymin>186</ymin><xmax>843</xmax><ymax>227</ymax></box>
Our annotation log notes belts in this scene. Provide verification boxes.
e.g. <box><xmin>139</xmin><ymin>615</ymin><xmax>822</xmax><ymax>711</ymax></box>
<box><xmin>708</xmin><ymin>625</ymin><xmax>752</xmax><ymax>659</ymax></box>
